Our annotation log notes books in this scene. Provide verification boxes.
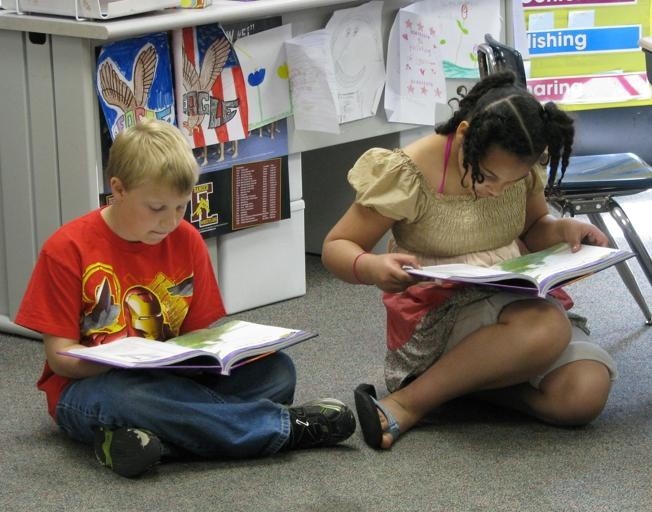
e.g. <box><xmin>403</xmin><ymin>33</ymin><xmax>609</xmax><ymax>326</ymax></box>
<box><xmin>399</xmin><ymin>236</ymin><xmax>642</xmax><ymax>301</ymax></box>
<box><xmin>57</xmin><ymin>313</ymin><xmax>318</xmax><ymax>378</ymax></box>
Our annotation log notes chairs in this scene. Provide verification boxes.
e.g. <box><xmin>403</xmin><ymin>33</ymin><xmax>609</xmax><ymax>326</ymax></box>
<box><xmin>477</xmin><ymin>33</ymin><xmax>652</xmax><ymax>326</ymax></box>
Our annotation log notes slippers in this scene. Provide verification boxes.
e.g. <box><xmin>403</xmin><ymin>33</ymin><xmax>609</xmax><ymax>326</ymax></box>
<box><xmin>355</xmin><ymin>384</ymin><xmax>401</xmax><ymax>449</ymax></box>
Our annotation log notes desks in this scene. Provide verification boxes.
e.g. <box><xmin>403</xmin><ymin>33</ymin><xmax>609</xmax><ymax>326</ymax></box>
<box><xmin>1</xmin><ymin>1</ymin><xmax>530</xmax><ymax>342</ymax></box>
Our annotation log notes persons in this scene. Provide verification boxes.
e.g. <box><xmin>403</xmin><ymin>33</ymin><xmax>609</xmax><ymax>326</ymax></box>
<box><xmin>317</xmin><ymin>67</ymin><xmax>620</xmax><ymax>453</ymax></box>
<box><xmin>8</xmin><ymin>117</ymin><xmax>359</xmax><ymax>478</ymax></box>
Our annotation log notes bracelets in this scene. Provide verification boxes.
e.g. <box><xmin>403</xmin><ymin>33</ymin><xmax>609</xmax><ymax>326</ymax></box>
<box><xmin>353</xmin><ymin>250</ymin><xmax>373</xmax><ymax>286</ymax></box>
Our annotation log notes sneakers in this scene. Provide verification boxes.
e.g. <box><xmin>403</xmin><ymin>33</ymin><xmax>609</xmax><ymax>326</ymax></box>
<box><xmin>286</xmin><ymin>396</ymin><xmax>356</xmax><ymax>446</ymax></box>
<box><xmin>94</xmin><ymin>427</ymin><xmax>167</xmax><ymax>478</ymax></box>
